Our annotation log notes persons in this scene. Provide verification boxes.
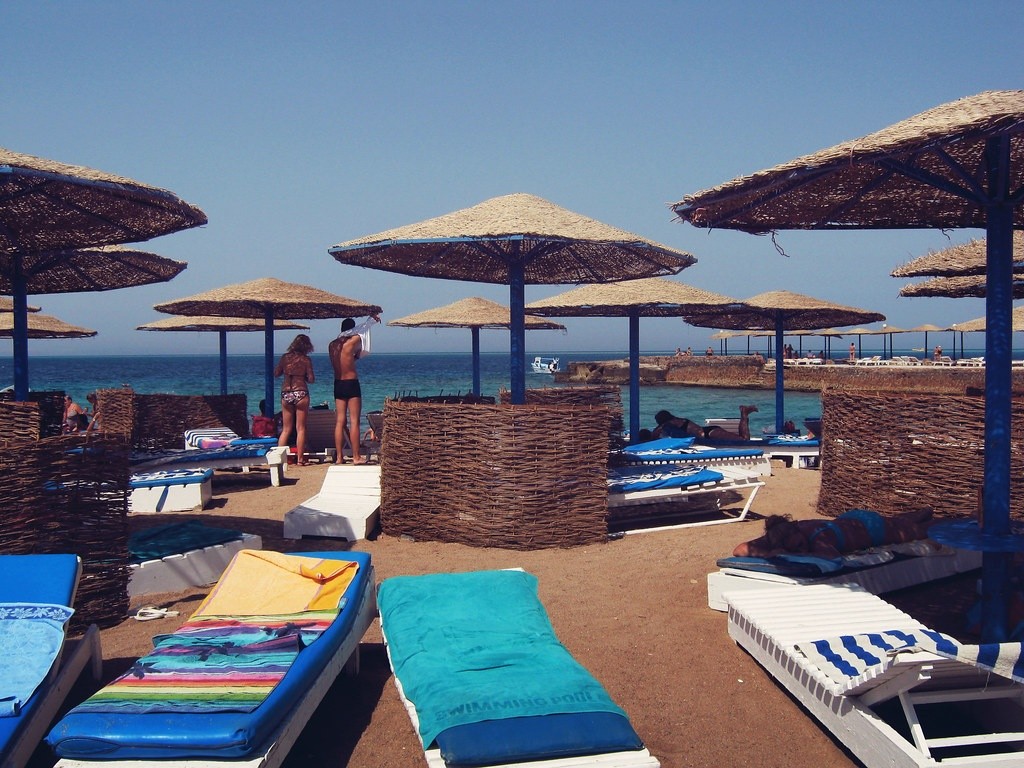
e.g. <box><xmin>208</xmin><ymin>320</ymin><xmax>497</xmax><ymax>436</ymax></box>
<box><xmin>621</xmin><ymin>405</ymin><xmax>758</xmax><ymax>442</ymax></box>
<box><xmin>329</xmin><ymin>312</ymin><xmax>379</xmax><ymax>465</ymax></box>
<box><xmin>275</xmin><ymin>335</ymin><xmax>315</xmax><ymax>467</ymax></box>
<box><xmin>934</xmin><ymin>345</ymin><xmax>942</xmax><ymax>362</ymax></box>
<box><xmin>706</xmin><ymin>347</ymin><xmax>713</xmax><ymax>356</ymax></box>
<box><xmin>733</xmin><ymin>505</ymin><xmax>961</xmax><ymax>559</ymax></box>
<box><xmin>783</xmin><ymin>344</ymin><xmax>824</xmax><ymax>359</ymax></box>
<box><xmin>850</xmin><ymin>342</ymin><xmax>856</xmax><ymax>361</ymax></box>
<box><xmin>675</xmin><ymin>347</ymin><xmax>692</xmax><ymax>356</ymax></box>
<box><xmin>63</xmin><ymin>392</ymin><xmax>99</xmax><ymax>433</ymax></box>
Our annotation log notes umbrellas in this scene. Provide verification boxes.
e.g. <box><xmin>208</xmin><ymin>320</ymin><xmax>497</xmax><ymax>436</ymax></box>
<box><xmin>684</xmin><ymin>288</ymin><xmax>887</xmax><ymax>435</ymax></box>
<box><xmin>131</xmin><ymin>277</ymin><xmax>384</xmax><ymax>415</ymax></box>
<box><xmin>814</xmin><ymin>327</ymin><xmax>873</xmax><ymax>359</ymax></box>
<box><xmin>870</xmin><ymin>324</ymin><xmax>964</xmax><ymax>358</ymax></box>
<box><xmin>784</xmin><ymin>329</ymin><xmax>813</xmax><ymax>358</ymax></box>
<box><xmin>0</xmin><ymin>146</ymin><xmax>208</xmax><ymax>401</ymax></box>
<box><xmin>387</xmin><ymin>296</ymin><xmax>565</xmax><ymax>393</ymax></box>
<box><xmin>674</xmin><ymin>85</ymin><xmax>1024</xmax><ymax>640</ymax></box>
<box><xmin>890</xmin><ymin>227</ymin><xmax>1024</xmax><ymax>331</ymax></box>
<box><xmin>522</xmin><ymin>277</ymin><xmax>749</xmax><ymax>445</ymax></box>
<box><xmin>709</xmin><ymin>330</ymin><xmax>776</xmax><ymax>358</ymax></box>
<box><xmin>327</xmin><ymin>190</ymin><xmax>697</xmax><ymax>405</ymax></box>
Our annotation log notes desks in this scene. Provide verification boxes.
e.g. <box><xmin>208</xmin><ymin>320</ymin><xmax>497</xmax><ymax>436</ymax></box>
<box><xmin>927</xmin><ymin>519</ymin><xmax>1024</xmax><ymax>552</ymax></box>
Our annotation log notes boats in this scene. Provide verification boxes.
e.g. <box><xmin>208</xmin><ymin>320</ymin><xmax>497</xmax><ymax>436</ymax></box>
<box><xmin>530</xmin><ymin>356</ymin><xmax>561</xmax><ymax>373</ymax></box>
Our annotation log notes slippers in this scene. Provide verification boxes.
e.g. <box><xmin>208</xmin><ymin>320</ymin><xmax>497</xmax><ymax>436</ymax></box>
<box><xmin>336</xmin><ymin>458</ymin><xmax>346</xmax><ymax>464</ymax></box>
<box><xmin>354</xmin><ymin>458</ymin><xmax>377</xmax><ymax>465</ymax></box>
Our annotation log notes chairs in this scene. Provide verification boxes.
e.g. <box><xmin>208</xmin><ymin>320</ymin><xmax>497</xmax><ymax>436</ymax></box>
<box><xmin>767</xmin><ymin>356</ymin><xmax>1024</xmax><ymax>365</ymax></box>
<box><xmin>0</xmin><ymin>409</ymin><xmax>1024</xmax><ymax>768</ymax></box>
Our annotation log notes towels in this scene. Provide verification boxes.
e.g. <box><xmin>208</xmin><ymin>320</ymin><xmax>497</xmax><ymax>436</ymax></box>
<box><xmin>184</xmin><ymin>427</ymin><xmax>241</xmax><ymax>447</ymax></box>
<box><xmin>64</xmin><ymin>549</ymin><xmax>360</xmax><ymax>714</ymax></box>
<box><xmin>377</xmin><ymin>569</ymin><xmax>629</xmax><ymax>753</ymax></box>
<box><xmin>610</xmin><ymin>444</ymin><xmax>703</xmax><ymax>454</ymax></box>
<box><xmin>796</xmin><ymin>628</ymin><xmax>1023</xmax><ymax>686</ymax></box>
<box><xmin>0</xmin><ymin>599</ymin><xmax>76</xmax><ymax>718</ymax></box>
<box><xmin>128</xmin><ymin>467</ymin><xmax>210</xmax><ymax>480</ymax></box>
<box><xmin>607</xmin><ymin>462</ymin><xmax>707</xmax><ymax>488</ymax></box>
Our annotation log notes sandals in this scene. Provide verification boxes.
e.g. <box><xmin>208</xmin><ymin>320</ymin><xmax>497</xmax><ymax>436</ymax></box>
<box><xmin>297</xmin><ymin>460</ymin><xmax>313</xmax><ymax>466</ymax></box>
